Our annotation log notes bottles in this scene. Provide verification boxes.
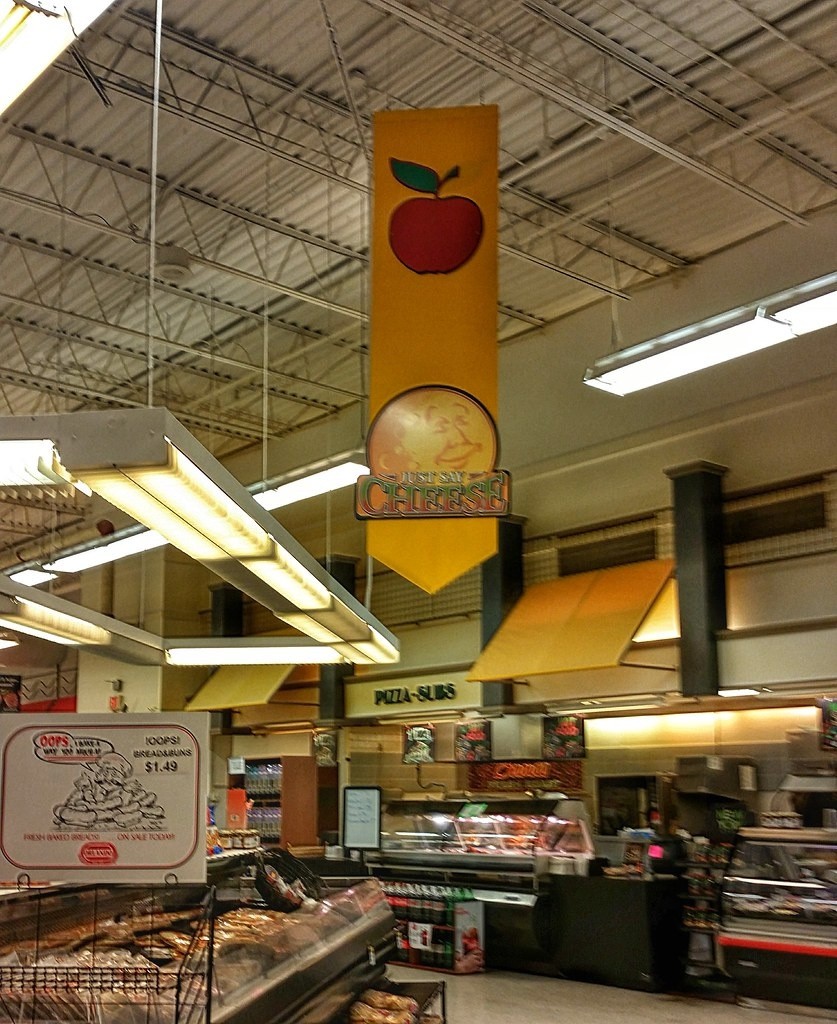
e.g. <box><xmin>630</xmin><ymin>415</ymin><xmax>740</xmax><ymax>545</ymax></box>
<box><xmin>378</xmin><ymin>881</ymin><xmax>473</xmax><ymax>969</ymax></box>
<box><xmin>246</xmin><ymin>763</ymin><xmax>282</xmax><ymax>844</ymax></box>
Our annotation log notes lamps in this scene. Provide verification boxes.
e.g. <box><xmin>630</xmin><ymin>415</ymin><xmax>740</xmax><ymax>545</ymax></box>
<box><xmin>0</xmin><ymin>410</ymin><xmax>404</xmax><ymax>672</ymax></box>
<box><xmin>584</xmin><ymin>270</ymin><xmax>837</xmax><ymax>397</ymax></box>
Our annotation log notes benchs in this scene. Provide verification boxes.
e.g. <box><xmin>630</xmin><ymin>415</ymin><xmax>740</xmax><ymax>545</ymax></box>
<box><xmin>389</xmin><ymin>979</ymin><xmax>446</xmax><ymax>1023</ymax></box>
